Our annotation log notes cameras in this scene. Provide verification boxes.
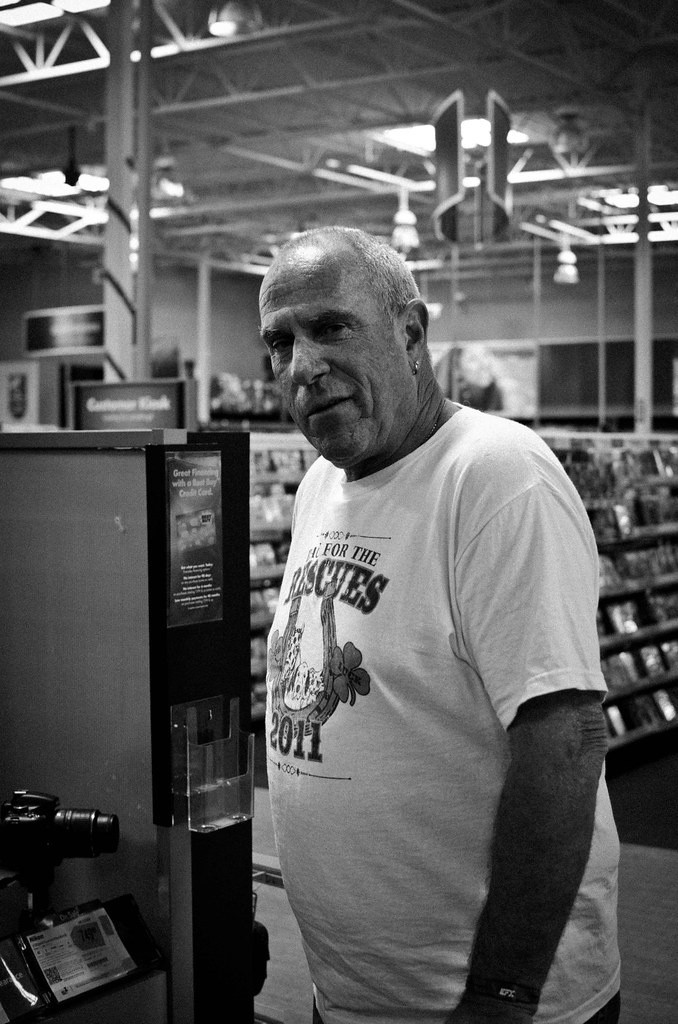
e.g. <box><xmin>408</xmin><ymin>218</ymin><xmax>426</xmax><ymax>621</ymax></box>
<box><xmin>0</xmin><ymin>788</ymin><xmax>121</xmax><ymax>872</ymax></box>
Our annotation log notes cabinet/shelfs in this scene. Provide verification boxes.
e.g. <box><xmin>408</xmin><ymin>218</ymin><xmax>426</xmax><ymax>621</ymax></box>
<box><xmin>187</xmin><ymin>366</ymin><xmax>678</xmax><ymax>838</ymax></box>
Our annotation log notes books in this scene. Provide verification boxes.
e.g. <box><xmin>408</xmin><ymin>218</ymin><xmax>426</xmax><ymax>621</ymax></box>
<box><xmin>249</xmin><ymin>444</ymin><xmax>324</xmax><ymax>716</ymax></box>
<box><xmin>566</xmin><ymin>436</ymin><xmax>678</xmax><ymax>744</ymax></box>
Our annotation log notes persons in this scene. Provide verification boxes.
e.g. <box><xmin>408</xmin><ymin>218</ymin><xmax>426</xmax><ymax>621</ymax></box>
<box><xmin>253</xmin><ymin>222</ymin><xmax>628</xmax><ymax>1024</ymax></box>
